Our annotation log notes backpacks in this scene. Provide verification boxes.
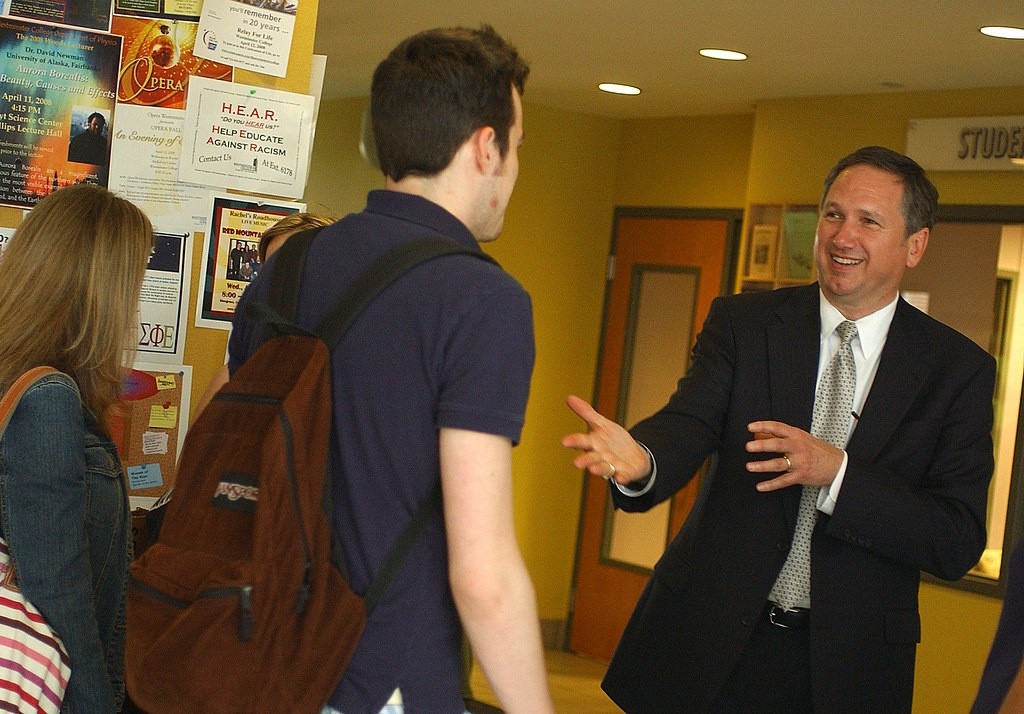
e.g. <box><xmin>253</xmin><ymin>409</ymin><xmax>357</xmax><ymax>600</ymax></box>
<box><xmin>125</xmin><ymin>229</ymin><xmax>504</xmax><ymax>714</ymax></box>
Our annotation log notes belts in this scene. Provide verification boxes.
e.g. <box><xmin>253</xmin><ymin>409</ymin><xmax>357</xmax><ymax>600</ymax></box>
<box><xmin>759</xmin><ymin>600</ymin><xmax>810</xmax><ymax>636</ymax></box>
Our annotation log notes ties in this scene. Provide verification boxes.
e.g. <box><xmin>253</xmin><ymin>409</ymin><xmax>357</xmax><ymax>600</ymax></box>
<box><xmin>770</xmin><ymin>321</ymin><xmax>858</xmax><ymax>614</ymax></box>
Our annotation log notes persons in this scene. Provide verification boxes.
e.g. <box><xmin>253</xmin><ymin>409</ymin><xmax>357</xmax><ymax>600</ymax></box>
<box><xmin>69</xmin><ymin>113</ymin><xmax>106</xmax><ymax>165</ymax></box>
<box><xmin>228</xmin><ymin>25</ymin><xmax>555</xmax><ymax>714</ymax></box>
<box><xmin>0</xmin><ymin>184</ymin><xmax>153</xmax><ymax>714</ymax></box>
<box><xmin>562</xmin><ymin>145</ymin><xmax>995</xmax><ymax>714</ymax></box>
<box><xmin>969</xmin><ymin>539</ymin><xmax>1024</xmax><ymax>714</ymax></box>
<box><xmin>259</xmin><ymin>213</ymin><xmax>340</xmax><ymax>269</ymax></box>
<box><xmin>231</xmin><ymin>242</ymin><xmax>260</xmax><ymax>281</ymax></box>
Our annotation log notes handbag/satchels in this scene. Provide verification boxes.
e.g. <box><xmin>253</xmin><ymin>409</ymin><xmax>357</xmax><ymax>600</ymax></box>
<box><xmin>0</xmin><ymin>364</ymin><xmax>82</xmax><ymax>714</ymax></box>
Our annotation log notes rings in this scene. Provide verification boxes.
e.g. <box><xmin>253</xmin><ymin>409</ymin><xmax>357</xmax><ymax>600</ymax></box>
<box><xmin>600</xmin><ymin>461</ymin><xmax>616</xmax><ymax>481</ymax></box>
<box><xmin>783</xmin><ymin>455</ymin><xmax>791</xmax><ymax>471</ymax></box>
